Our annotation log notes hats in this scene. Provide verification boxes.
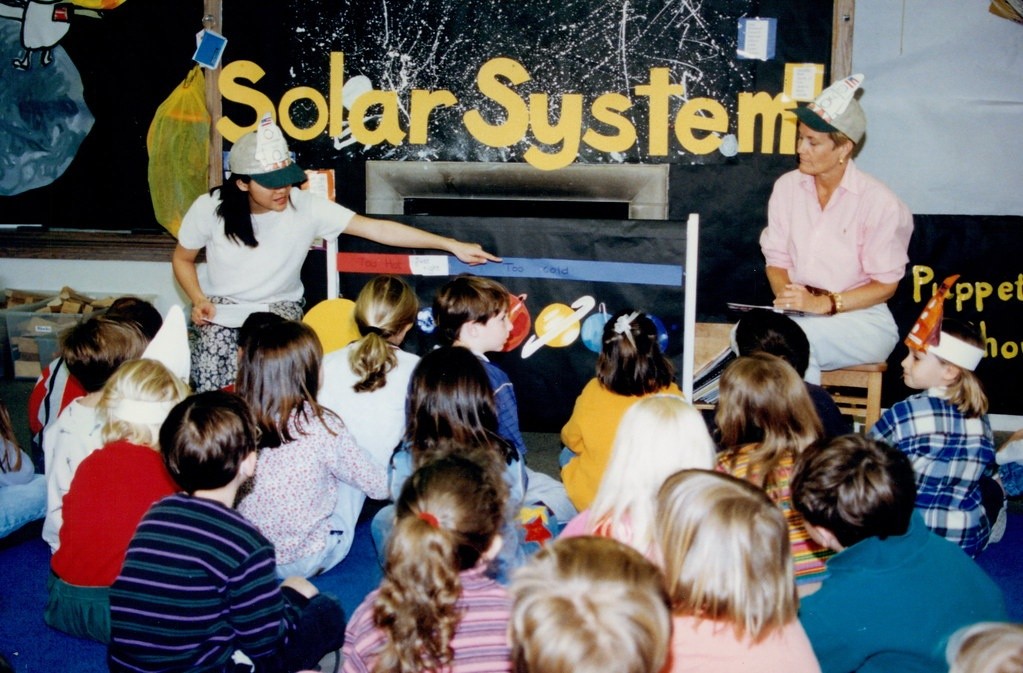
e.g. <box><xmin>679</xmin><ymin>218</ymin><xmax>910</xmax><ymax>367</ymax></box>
<box><xmin>228</xmin><ymin>113</ymin><xmax>307</xmax><ymax>188</ymax></box>
<box><xmin>783</xmin><ymin>73</ymin><xmax>866</xmax><ymax>144</ymax></box>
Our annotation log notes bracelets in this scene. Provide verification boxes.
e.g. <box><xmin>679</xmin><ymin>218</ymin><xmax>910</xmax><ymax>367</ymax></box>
<box><xmin>829</xmin><ymin>293</ymin><xmax>842</xmax><ymax>315</ymax></box>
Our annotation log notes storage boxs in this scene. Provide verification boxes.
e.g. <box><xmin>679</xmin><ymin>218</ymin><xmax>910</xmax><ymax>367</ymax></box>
<box><xmin>0</xmin><ymin>290</ymin><xmax>156</xmax><ymax>379</ymax></box>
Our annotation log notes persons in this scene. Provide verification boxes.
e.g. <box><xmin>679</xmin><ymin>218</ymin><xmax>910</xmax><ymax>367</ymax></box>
<box><xmin>108</xmin><ymin>391</ymin><xmax>346</xmax><ymax>673</ymax></box>
<box><xmin>506</xmin><ymin>535</ymin><xmax>675</xmax><ymax>673</ymax></box>
<box><xmin>715</xmin><ymin>352</ymin><xmax>846</xmax><ymax>616</ymax></box>
<box><xmin>44</xmin><ymin>358</ymin><xmax>192</xmax><ymax>646</ymax></box>
<box><xmin>171</xmin><ymin>113</ymin><xmax>502</xmax><ymax>394</ymax></box>
<box><xmin>341</xmin><ymin>456</ymin><xmax>522</xmax><ymax>673</ymax></box>
<box><xmin>559</xmin><ymin>310</ymin><xmax>687</xmax><ymax>514</ymax></box>
<box><xmin>951</xmin><ymin>622</ymin><xmax>1023</xmax><ymax>673</ymax></box>
<box><xmin>557</xmin><ymin>395</ymin><xmax>725</xmax><ymax>576</ymax></box>
<box><xmin>431</xmin><ymin>273</ymin><xmax>529</xmax><ymax>467</ymax></box>
<box><xmin>865</xmin><ymin>316</ymin><xmax>1003</xmax><ymax>561</ymax></box>
<box><xmin>220</xmin><ymin>312</ymin><xmax>389</xmax><ymax>580</ymax></box>
<box><xmin>788</xmin><ymin>434</ymin><xmax>1010</xmax><ymax>673</ymax></box>
<box><xmin>29</xmin><ymin>296</ymin><xmax>163</xmax><ymax>471</ymax></box>
<box><xmin>651</xmin><ymin>467</ymin><xmax>820</xmax><ymax>673</ymax></box>
<box><xmin>0</xmin><ymin>392</ymin><xmax>46</xmax><ymax>537</ymax></box>
<box><xmin>41</xmin><ymin>313</ymin><xmax>150</xmax><ymax>556</ymax></box>
<box><xmin>759</xmin><ymin>95</ymin><xmax>914</xmax><ymax>387</ymax></box>
<box><xmin>318</xmin><ymin>276</ymin><xmax>422</xmax><ymax>519</ymax></box>
<box><xmin>370</xmin><ymin>345</ymin><xmax>558</xmax><ymax>585</ymax></box>
<box><xmin>713</xmin><ymin>307</ymin><xmax>852</xmax><ymax>452</ymax></box>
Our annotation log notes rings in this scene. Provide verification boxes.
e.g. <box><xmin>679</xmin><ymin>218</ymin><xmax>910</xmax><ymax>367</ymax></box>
<box><xmin>787</xmin><ymin>304</ymin><xmax>792</xmax><ymax>309</ymax></box>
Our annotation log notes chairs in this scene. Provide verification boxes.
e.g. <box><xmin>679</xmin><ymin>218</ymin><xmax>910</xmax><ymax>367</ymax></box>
<box><xmin>819</xmin><ymin>361</ymin><xmax>888</xmax><ymax>436</ymax></box>
